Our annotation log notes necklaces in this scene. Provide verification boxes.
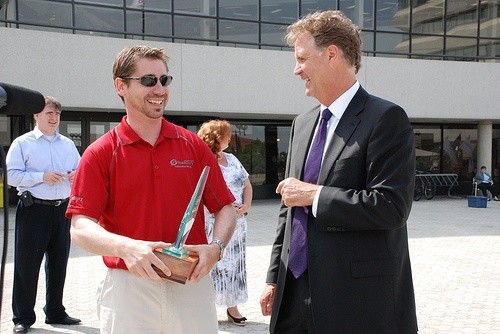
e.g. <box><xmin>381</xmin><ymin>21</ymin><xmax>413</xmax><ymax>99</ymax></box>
<box><xmin>218</xmin><ymin>151</ymin><xmax>223</xmax><ymax>160</ymax></box>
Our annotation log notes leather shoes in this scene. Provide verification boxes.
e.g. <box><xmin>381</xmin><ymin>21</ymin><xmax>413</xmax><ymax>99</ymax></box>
<box><xmin>13</xmin><ymin>323</ymin><xmax>29</xmax><ymax>334</ymax></box>
<box><xmin>45</xmin><ymin>314</ymin><xmax>82</xmax><ymax>324</ymax></box>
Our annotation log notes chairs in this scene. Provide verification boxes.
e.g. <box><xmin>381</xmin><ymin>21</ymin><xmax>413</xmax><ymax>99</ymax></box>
<box><xmin>472</xmin><ymin>177</ymin><xmax>493</xmax><ymax>199</ymax></box>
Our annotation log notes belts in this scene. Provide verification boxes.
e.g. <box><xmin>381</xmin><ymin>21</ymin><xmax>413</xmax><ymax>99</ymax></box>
<box><xmin>33</xmin><ymin>198</ymin><xmax>69</xmax><ymax>206</ymax></box>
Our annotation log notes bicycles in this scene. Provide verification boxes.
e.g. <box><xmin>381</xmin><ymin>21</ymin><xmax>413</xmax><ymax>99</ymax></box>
<box><xmin>413</xmin><ymin>169</ymin><xmax>436</xmax><ymax>200</ymax></box>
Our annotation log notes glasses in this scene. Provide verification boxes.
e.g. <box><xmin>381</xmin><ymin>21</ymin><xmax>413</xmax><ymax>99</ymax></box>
<box><xmin>120</xmin><ymin>74</ymin><xmax>172</xmax><ymax>87</ymax></box>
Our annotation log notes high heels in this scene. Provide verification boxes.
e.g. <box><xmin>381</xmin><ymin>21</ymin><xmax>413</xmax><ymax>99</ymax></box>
<box><xmin>227</xmin><ymin>308</ymin><xmax>247</xmax><ymax>326</ymax></box>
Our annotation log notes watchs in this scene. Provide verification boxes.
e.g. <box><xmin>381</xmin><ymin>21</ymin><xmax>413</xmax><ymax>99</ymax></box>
<box><xmin>210</xmin><ymin>239</ymin><xmax>226</xmax><ymax>261</ymax></box>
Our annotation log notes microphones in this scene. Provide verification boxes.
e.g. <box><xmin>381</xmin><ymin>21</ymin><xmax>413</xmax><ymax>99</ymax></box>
<box><xmin>0</xmin><ymin>82</ymin><xmax>45</xmax><ymax>114</ymax></box>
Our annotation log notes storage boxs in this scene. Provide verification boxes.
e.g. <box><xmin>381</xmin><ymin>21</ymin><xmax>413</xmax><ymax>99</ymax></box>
<box><xmin>151</xmin><ymin>247</ymin><xmax>199</xmax><ymax>285</ymax></box>
<box><xmin>466</xmin><ymin>196</ymin><xmax>488</xmax><ymax>208</ymax></box>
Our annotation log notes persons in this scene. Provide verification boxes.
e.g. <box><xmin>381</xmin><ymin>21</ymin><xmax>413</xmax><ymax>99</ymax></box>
<box><xmin>472</xmin><ymin>163</ymin><xmax>500</xmax><ymax>200</ymax></box>
<box><xmin>195</xmin><ymin>120</ymin><xmax>253</xmax><ymax>326</ymax></box>
<box><xmin>258</xmin><ymin>10</ymin><xmax>419</xmax><ymax>334</ymax></box>
<box><xmin>5</xmin><ymin>97</ymin><xmax>82</xmax><ymax>334</ymax></box>
<box><xmin>65</xmin><ymin>45</ymin><xmax>238</xmax><ymax>334</ymax></box>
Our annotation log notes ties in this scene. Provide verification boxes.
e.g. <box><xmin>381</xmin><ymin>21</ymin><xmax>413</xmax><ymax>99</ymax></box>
<box><xmin>287</xmin><ymin>108</ymin><xmax>334</xmax><ymax>280</ymax></box>
